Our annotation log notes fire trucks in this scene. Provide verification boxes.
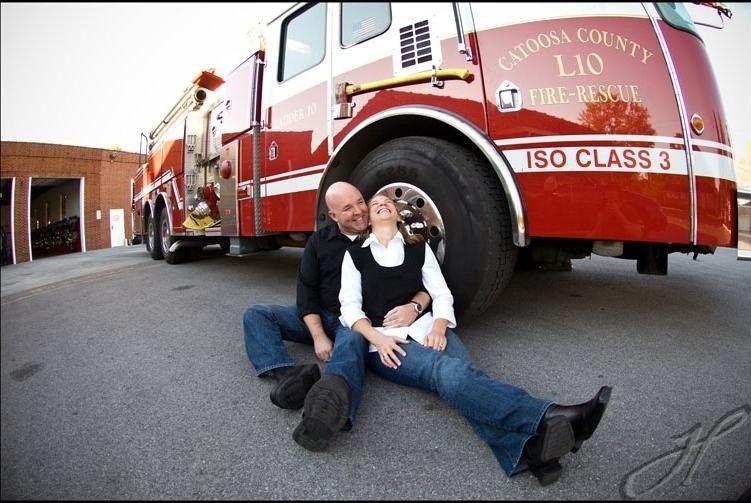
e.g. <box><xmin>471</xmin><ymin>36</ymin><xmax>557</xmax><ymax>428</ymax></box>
<box><xmin>134</xmin><ymin>2</ymin><xmax>738</xmax><ymax>327</ymax></box>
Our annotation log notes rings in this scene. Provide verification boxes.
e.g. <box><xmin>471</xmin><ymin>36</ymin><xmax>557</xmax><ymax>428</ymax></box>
<box><xmin>438</xmin><ymin>342</ymin><xmax>444</xmax><ymax>348</ymax></box>
<box><xmin>383</xmin><ymin>352</ymin><xmax>388</xmax><ymax>358</ymax></box>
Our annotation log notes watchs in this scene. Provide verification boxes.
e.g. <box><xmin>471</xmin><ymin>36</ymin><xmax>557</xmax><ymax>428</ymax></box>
<box><xmin>408</xmin><ymin>299</ymin><xmax>424</xmax><ymax>316</ymax></box>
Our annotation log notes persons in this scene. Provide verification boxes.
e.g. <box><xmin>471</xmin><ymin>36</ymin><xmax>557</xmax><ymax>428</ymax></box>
<box><xmin>242</xmin><ymin>180</ymin><xmax>434</xmax><ymax>452</ymax></box>
<box><xmin>338</xmin><ymin>192</ymin><xmax>616</xmax><ymax>490</ymax></box>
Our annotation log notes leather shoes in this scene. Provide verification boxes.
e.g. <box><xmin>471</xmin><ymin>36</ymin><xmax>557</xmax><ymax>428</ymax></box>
<box><xmin>267</xmin><ymin>363</ymin><xmax>319</xmax><ymax>412</ymax></box>
<box><xmin>292</xmin><ymin>375</ymin><xmax>348</xmax><ymax>452</ymax></box>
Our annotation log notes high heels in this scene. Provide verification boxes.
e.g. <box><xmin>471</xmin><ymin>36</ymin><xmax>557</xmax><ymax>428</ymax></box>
<box><xmin>525</xmin><ymin>415</ymin><xmax>577</xmax><ymax>491</ymax></box>
<box><xmin>544</xmin><ymin>384</ymin><xmax>613</xmax><ymax>451</ymax></box>
